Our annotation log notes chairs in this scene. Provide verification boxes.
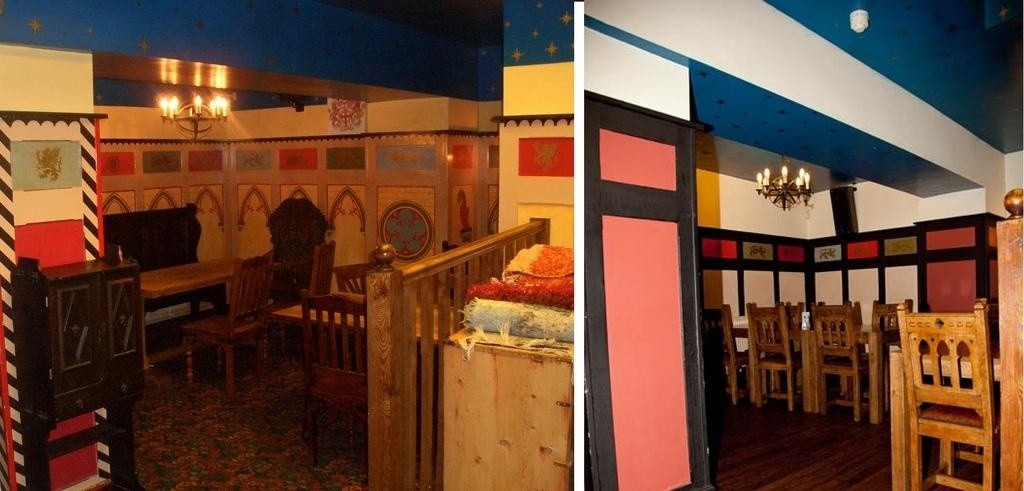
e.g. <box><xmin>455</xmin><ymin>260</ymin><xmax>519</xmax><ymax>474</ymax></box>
<box><xmin>893</xmin><ymin>300</ymin><xmax>1000</xmax><ymax>490</ymax></box>
<box><xmin>252</xmin><ymin>197</ymin><xmax>327</xmax><ymax>312</ymax></box>
<box><xmin>440</xmin><ymin>236</ymin><xmax>468</xmax><ymax>307</ymax></box>
<box><xmin>182</xmin><ymin>255</ymin><xmax>272</xmax><ymax>395</ymax></box>
<box><xmin>309</xmin><ymin>240</ymin><xmax>335</xmax><ymax>294</ymax></box>
<box><xmin>331</xmin><ymin>262</ymin><xmax>376</xmax><ymax>295</ymax></box>
<box><xmin>300</xmin><ymin>289</ymin><xmax>366</xmax><ymax>466</ymax></box>
<box><xmin>704</xmin><ymin>300</ymin><xmax>910</xmax><ymax>425</ymax></box>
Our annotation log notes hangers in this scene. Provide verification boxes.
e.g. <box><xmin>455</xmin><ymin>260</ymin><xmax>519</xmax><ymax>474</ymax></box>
<box><xmin>271</xmin><ymin>295</ymin><xmax>453</xmax><ymax>365</ymax></box>
<box><xmin>886</xmin><ymin>343</ymin><xmax>999</xmax><ymax>490</ymax></box>
<box><xmin>139</xmin><ymin>256</ymin><xmax>240</xmax><ymax>369</ymax></box>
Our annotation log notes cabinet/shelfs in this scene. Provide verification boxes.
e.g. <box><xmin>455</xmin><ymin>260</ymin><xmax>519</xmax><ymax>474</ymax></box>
<box><xmin>441</xmin><ymin>329</ymin><xmax>573</xmax><ymax>491</ymax></box>
<box><xmin>12</xmin><ymin>246</ymin><xmax>145</xmax><ymax>491</ymax></box>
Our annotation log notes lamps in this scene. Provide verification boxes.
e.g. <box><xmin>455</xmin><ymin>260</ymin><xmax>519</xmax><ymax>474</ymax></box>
<box><xmin>157</xmin><ymin>91</ymin><xmax>228</xmax><ymax>139</ymax></box>
<box><xmin>756</xmin><ymin>156</ymin><xmax>813</xmax><ymax>211</ymax></box>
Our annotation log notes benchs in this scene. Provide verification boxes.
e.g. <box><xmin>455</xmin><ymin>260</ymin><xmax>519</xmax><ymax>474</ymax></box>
<box><xmin>104</xmin><ymin>204</ymin><xmax>227</xmax><ymax>345</ymax></box>
